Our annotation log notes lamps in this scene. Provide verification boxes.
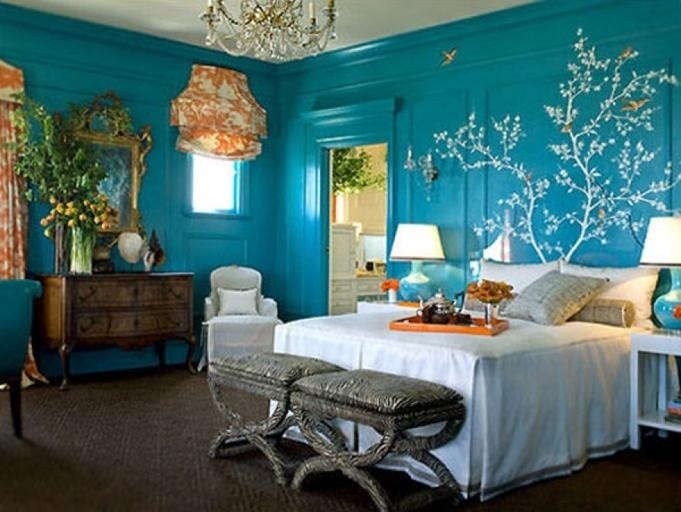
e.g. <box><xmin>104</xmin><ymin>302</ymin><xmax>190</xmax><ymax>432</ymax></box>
<box><xmin>637</xmin><ymin>211</ymin><xmax>681</xmax><ymax>332</ymax></box>
<box><xmin>388</xmin><ymin>224</ymin><xmax>446</xmax><ymax>304</ymax></box>
<box><xmin>195</xmin><ymin>2</ymin><xmax>345</xmax><ymax>67</ymax></box>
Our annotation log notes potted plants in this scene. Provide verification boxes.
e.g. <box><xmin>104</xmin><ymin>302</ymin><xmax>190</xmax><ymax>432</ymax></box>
<box><xmin>10</xmin><ymin>84</ymin><xmax>122</xmax><ymax>276</ymax></box>
<box><xmin>326</xmin><ymin>149</ymin><xmax>383</xmax><ymax>224</ymax></box>
<box><xmin>382</xmin><ymin>277</ymin><xmax>402</xmax><ymax>302</ymax></box>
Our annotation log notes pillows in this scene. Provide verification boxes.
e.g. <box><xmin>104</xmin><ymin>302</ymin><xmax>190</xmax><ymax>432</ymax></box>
<box><xmin>477</xmin><ymin>260</ymin><xmax>559</xmax><ymax>321</ymax></box>
<box><xmin>570</xmin><ymin>296</ymin><xmax>636</xmax><ymax>328</ymax></box>
<box><xmin>217</xmin><ymin>287</ymin><xmax>260</xmax><ymax>316</ymax></box>
<box><xmin>496</xmin><ymin>269</ymin><xmax>608</xmax><ymax>326</ymax></box>
<box><xmin>558</xmin><ymin>260</ymin><xmax>658</xmax><ymax>332</ymax></box>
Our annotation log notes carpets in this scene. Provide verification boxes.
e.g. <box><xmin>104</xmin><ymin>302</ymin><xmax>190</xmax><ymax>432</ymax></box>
<box><xmin>0</xmin><ymin>363</ymin><xmax>681</xmax><ymax>512</ymax></box>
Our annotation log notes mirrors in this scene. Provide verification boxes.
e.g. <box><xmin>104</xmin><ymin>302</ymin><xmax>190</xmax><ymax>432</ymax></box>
<box><xmin>52</xmin><ymin>90</ymin><xmax>155</xmax><ymax>274</ymax></box>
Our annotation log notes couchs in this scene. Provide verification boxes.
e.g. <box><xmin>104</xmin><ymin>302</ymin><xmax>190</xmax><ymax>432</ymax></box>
<box><xmin>198</xmin><ymin>261</ymin><xmax>286</xmax><ymax>373</ymax></box>
<box><xmin>0</xmin><ymin>277</ymin><xmax>44</xmax><ymax>443</ymax></box>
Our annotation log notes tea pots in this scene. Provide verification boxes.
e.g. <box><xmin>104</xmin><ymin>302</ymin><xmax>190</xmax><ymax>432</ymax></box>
<box><xmin>418</xmin><ymin>289</ymin><xmax>465</xmax><ymax>324</ymax></box>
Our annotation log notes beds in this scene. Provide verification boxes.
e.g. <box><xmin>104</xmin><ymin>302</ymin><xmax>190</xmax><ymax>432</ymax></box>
<box><xmin>274</xmin><ymin>258</ymin><xmax>661</xmax><ymax>511</ymax></box>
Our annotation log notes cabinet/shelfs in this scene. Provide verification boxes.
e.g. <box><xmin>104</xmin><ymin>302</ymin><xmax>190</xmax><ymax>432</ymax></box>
<box><xmin>29</xmin><ymin>271</ymin><xmax>200</xmax><ymax>388</ymax></box>
<box><xmin>329</xmin><ymin>225</ymin><xmax>359</xmax><ymax>316</ymax></box>
<box><xmin>356</xmin><ymin>273</ymin><xmax>387</xmax><ymax>297</ymax></box>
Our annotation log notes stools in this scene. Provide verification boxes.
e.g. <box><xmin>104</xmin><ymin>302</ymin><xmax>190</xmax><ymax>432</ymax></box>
<box><xmin>290</xmin><ymin>370</ymin><xmax>468</xmax><ymax>512</ymax></box>
<box><xmin>209</xmin><ymin>350</ymin><xmax>344</xmax><ymax>493</ymax></box>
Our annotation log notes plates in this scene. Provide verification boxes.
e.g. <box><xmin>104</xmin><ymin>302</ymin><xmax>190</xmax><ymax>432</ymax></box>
<box><xmin>390</xmin><ymin>315</ymin><xmax>508</xmax><ymax>336</ymax></box>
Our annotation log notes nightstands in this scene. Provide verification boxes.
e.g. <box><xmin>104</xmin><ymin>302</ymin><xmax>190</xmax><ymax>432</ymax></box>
<box><xmin>631</xmin><ymin>333</ymin><xmax>681</xmax><ymax>450</ymax></box>
